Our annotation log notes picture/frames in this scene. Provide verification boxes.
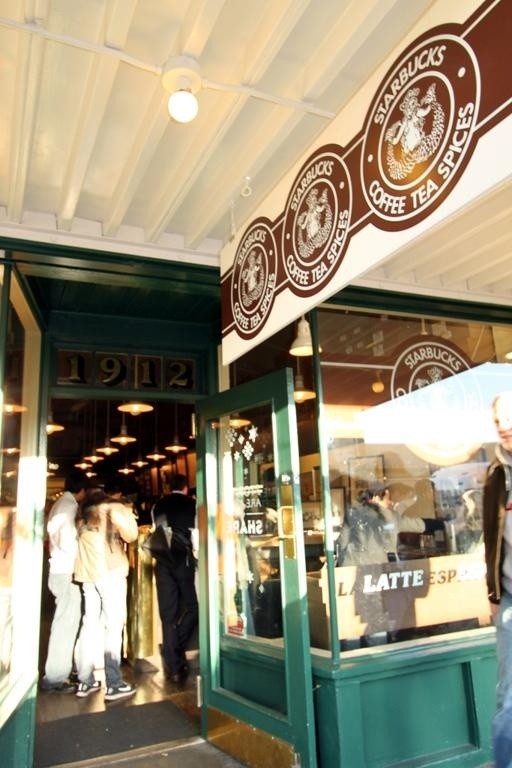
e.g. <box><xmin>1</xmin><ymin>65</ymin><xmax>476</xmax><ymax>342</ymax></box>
<box><xmin>298</xmin><ymin>486</ymin><xmax>346</xmax><ymax>529</ymax></box>
<box><xmin>348</xmin><ymin>454</ymin><xmax>387</xmax><ymax>511</ymax></box>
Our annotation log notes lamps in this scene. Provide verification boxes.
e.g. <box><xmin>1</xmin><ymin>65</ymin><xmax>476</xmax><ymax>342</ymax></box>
<box><xmin>159</xmin><ymin>54</ymin><xmax>204</xmax><ymax>124</ymax></box>
<box><xmin>371</xmin><ymin>368</ymin><xmax>385</xmax><ymax>394</ymax></box>
<box><xmin>287</xmin><ymin>312</ymin><xmax>322</xmax><ymax>404</ymax></box>
<box><xmin>226</xmin><ymin>359</ymin><xmax>252</xmax><ymax>429</ymax></box>
<box><xmin>75</xmin><ymin>398</ymin><xmax>186</xmax><ymax>477</ymax></box>
<box><xmin>1</xmin><ymin>393</ymin><xmax>65</xmax><ymax>480</ymax></box>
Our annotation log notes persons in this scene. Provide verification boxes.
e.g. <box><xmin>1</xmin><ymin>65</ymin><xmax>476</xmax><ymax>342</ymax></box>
<box><xmin>480</xmin><ymin>392</ymin><xmax>512</xmax><ymax>768</ymax></box>
<box><xmin>41</xmin><ymin>467</ymin><xmax>199</xmax><ymax>700</ymax></box>
<box><xmin>249</xmin><ymin>469</ymin><xmax>281</xmax><ymax>637</ymax></box>
<box><xmin>333</xmin><ymin>482</ymin><xmax>458</xmax><ymax>567</ymax></box>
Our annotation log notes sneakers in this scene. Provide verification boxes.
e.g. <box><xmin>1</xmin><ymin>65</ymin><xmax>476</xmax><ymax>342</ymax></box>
<box><xmin>105</xmin><ymin>678</ymin><xmax>136</xmax><ymax>700</ymax></box>
<box><xmin>75</xmin><ymin>681</ymin><xmax>102</xmax><ymax>697</ymax></box>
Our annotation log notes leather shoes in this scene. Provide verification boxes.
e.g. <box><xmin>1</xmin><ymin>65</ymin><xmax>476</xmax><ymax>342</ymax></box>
<box><xmin>41</xmin><ymin>678</ymin><xmax>76</xmax><ymax>694</ymax></box>
<box><xmin>171</xmin><ymin>665</ymin><xmax>190</xmax><ymax>683</ymax></box>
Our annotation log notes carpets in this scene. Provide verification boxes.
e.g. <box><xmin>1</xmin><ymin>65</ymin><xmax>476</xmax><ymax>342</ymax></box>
<box><xmin>34</xmin><ymin>698</ymin><xmax>197</xmax><ymax>768</ymax></box>
